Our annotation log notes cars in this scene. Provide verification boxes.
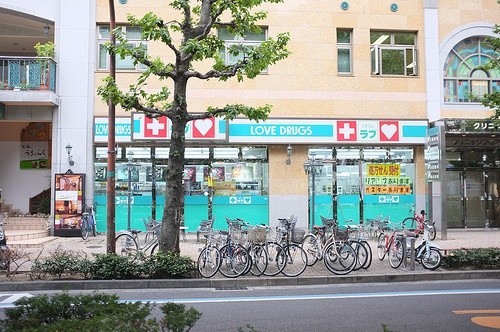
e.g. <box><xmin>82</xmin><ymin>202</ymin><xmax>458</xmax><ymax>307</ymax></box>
<box><xmin>0</xmin><ymin>211</ymin><xmax>12</xmax><ymax>269</ymax></box>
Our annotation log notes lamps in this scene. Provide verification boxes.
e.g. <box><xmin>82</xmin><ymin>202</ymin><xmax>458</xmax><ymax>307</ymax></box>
<box><xmin>65</xmin><ymin>142</ymin><xmax>74</xmax><ymax>166</ymax></box>
<box><xmin>286</xmin><ymin>144</ymin><xmax>293</xmax><ymax>164</ymax></box>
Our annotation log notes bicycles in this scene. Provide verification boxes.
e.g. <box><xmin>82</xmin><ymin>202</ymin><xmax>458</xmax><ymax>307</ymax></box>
<box><xmin>114</xmin><ymin>224</ymin><xmax>160</xmax><ymax>262</ymax></box>
<box><xmin>80</xmin><ymin>202</ymin><xmax>99</xmax><ymax>240</ymax></box>
<box><xmin>197</xmin><ymin>204</ymin><xmax>443</xmax><ymax>278</ymax></box>
<box><xmin>402</xmin><ymin>204</ymin><xmax>436</xmax><ymax>241</ymax></box>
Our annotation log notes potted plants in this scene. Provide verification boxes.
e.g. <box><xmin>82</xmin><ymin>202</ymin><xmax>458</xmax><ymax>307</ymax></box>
<box><xmin>35</xmin><ymin>41</ymin><xmax>56</xmax><ymax>90</ymax></box>
